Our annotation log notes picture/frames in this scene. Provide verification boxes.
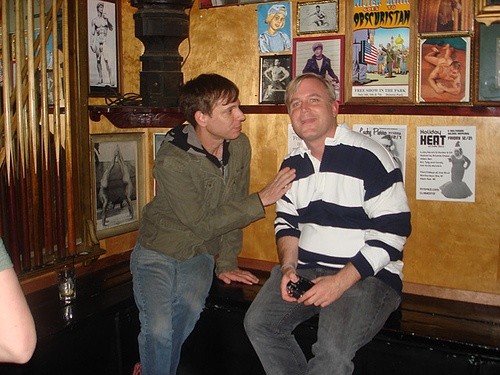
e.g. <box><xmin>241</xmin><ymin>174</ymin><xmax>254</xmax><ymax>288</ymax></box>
<box><xmin>417</xmin><ymin>31</ymin><xmax>474</xmax><ymax>107</ymax></box>
<box><xmin>258</xmin><ymin>54</ymin><xmax>291</xmax><ymax>104</ymax></box>
<box><xmin>86</xmin><ymin>0</ymin><xmax>122</xmax><ymax>98</ymax></box>
<box><xmin>344</xmin><ymin>0</ymin><xmax>417</xmax><ymax>106</ymax></box>
<box><xmin>469</xmin><ymin>17</ymin><xmax>500</xmax><ymax>107</ymax></box>
<box><xmin>148</xmin><ymin>127</ymin><xmax>173</xmax><ymax>201</ymax></box>
<box><xmin>88</xmin><ymin>132</ymin><xmax>148</xmax><ymax>240</ymax></box>
<box><xmin>291</xmin><ymin>34</ymin><xmax>344</xmax><ymax>105</ymax></box>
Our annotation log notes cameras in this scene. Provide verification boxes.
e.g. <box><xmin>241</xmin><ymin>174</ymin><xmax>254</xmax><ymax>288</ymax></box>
<box><xmin>287</xmin><ymin>275</ymin><xmax>315</xmax><ymax>298</ymax></box>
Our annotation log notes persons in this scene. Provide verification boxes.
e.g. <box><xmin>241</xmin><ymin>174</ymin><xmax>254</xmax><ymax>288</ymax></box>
<box><xmin>0</xmin><ymin>240</ymin><xmax>37</xmax><ymax>364</ymax></box>
<box><xmin>99</xmin><ymin>145</ymin><xmax>134</xmax><ymax>225</ymax></box>
<box><xmin>302</xmin><ymin>42</ymin><xmax>339</xmax><ymax>87</ymax></box>
<box><xmin>90</xmin><ymin>2</ymin><xmax>113</xmax><ymax>85</ymax></box>
<box><xmin>424</xmin><ymin>43</ymin><xmax>461</xmax><ymax>94</ymax></box>
<box><xmin>244</xmin><ymin>73</ymin><xmax>412</xmax><ymax>375</ymax></box>
<box><xmin>263</xmin><ymin>59</ymin><xmax>289</xmax><ymax>99</ymax></box>
<box><xmin>130</xmin><ymin>73</ymin><xmax>296</xmax><ymax>375</ymax></box>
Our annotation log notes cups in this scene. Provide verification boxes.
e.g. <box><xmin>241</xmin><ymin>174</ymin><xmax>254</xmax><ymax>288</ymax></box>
<box><xmin>57</xmin><ymin>269</ymin><xmax>77</xmax><ymax>304</ymax></box>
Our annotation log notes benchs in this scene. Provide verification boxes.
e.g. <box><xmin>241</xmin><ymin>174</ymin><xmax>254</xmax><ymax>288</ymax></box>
<box><xmin>25</xmin><ymin>258</ymin><xmax>500</xmax><ymax>375</ymax></box>
